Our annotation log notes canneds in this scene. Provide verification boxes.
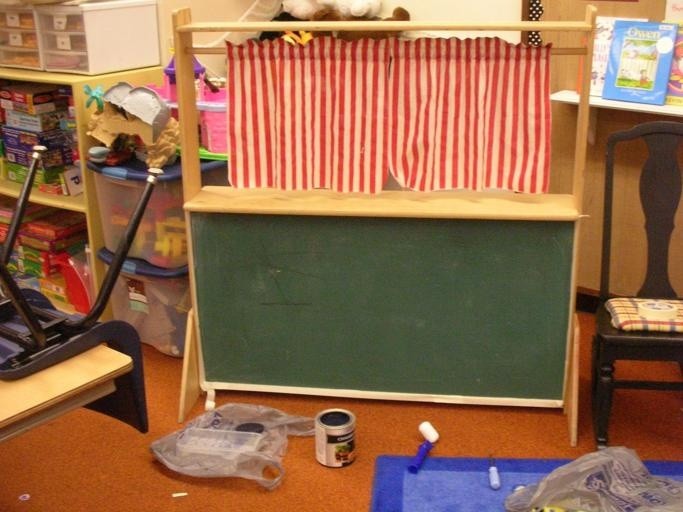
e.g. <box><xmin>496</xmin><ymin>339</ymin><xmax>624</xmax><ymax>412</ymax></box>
<box><xmin>314</xmin><ymin>408</ymin><xmax>356</xmax><ymax>468</ymax></box>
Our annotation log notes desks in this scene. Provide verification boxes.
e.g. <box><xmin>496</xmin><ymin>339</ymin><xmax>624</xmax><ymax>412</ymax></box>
<box><xmin>0</xmin><ymin>342</ymin><xmax>135</xmax><ymax>449</ymax></box>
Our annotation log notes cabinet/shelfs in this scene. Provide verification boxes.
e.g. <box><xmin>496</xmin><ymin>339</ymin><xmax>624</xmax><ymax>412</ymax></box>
<box><xmin>0</xmin><ymin>67</ymin><xmax>164</xmax><ymax>325</ymax></box>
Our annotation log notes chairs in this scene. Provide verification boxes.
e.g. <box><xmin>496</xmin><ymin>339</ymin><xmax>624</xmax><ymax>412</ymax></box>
<box><xmin>2</xmin><ymin>139</ymin><xmax>162</xmax><ymax>436</ymax></box>
<box><xmin>593</xmin><ymin>120</ymin><xmax>682</xmax><ymax>453</ymax></box>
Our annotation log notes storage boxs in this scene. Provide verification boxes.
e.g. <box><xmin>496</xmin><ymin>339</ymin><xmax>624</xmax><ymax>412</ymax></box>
<box><xmin>1</xmin><ymin>0</ymin><xmax>163</xmax><ymax>75</ymax></box>
<box><xmin>87</xmin><ymin>158</ymin><xmax>230</xmax><ymax>358</ymax></box>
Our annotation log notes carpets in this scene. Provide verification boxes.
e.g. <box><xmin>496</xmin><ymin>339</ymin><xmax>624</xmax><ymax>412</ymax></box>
<box><xmin>365</xmin><ymin>448</ymin><xmax>682</xmax><ymax>512</ymax></box>
